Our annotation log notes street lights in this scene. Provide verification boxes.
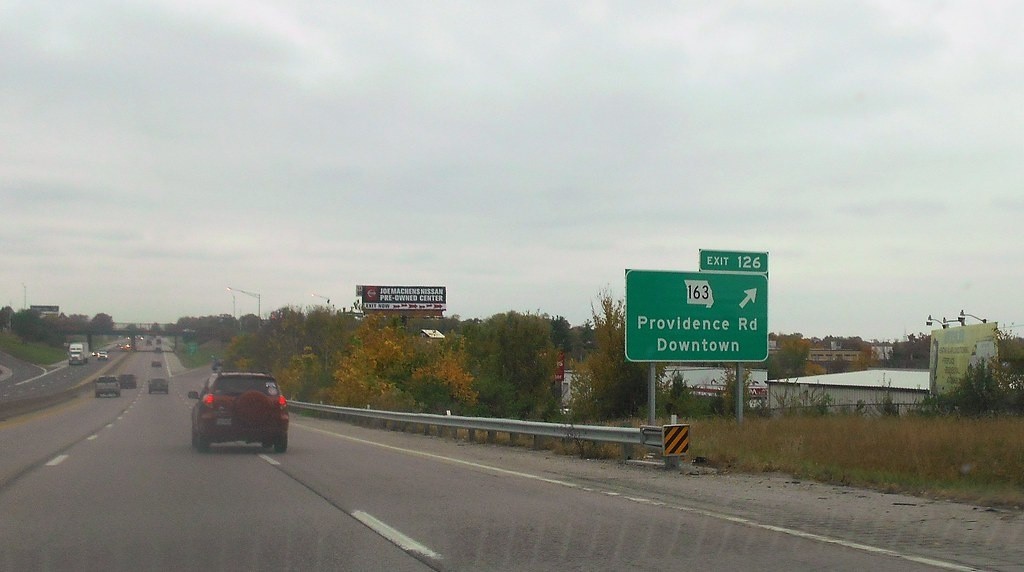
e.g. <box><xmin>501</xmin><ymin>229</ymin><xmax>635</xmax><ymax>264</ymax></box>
<box><xmin>225</xmin><ymin>286</ymin><xmax>263</xmax><ymax>318</ymax></box>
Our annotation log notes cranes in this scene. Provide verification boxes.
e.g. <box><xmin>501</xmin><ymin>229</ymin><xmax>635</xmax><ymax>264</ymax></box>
<box><xmin>311</xmin><ymin>294</ymin><xmax>331</xmax><ymax>305</ymax></box>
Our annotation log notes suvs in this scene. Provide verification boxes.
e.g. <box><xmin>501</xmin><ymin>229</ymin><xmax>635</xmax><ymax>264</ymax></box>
<box><xmin>186</xmin><ymin>371</ymin><xmax>289</xmax><ymax>453</ymax></box>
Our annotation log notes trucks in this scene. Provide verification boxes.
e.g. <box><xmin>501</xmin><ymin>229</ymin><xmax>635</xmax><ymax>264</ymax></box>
<box><xmin>69</xmin><ymin>341</ymin><xmax>90</xmax><ymax>365</ymax></box>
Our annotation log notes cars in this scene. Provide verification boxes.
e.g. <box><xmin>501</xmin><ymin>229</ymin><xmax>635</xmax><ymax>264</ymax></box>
<box><xmin>97</xmin><ymin>351</ymin><xmax>108</xmax><ymax>360</ymax></box>
<box><xmin>155</xmin><ymin>346</ymin><xmax>163</xmax><ymax>353</ymax></box>
<box><xmin>92</xmin><ymin>351</ymin><xmax>100</xmax><ymax>356</ymax></box>
<box><xmin>151</xmin><ymin>361</ymin><xmax>162</xmax><ymax>367</ymax></box>
<box><xmin>115</xmin><ymin>335</ymin><xmax>162</xmax><ymax>351</ymax></box>
<box><xmin>147</xmin><ymin>378</ymin><xmax>169</xmax><ymax>393</ymax></box>
<box><xmin>94</xmin><ymin>375</ymin><xmax>122</xmax><ymax>397</ymax></box>
<box><xmin>117</xmin><ymin>374</ymin><xmax>137</xmax><ymax>389</ymax></box>
